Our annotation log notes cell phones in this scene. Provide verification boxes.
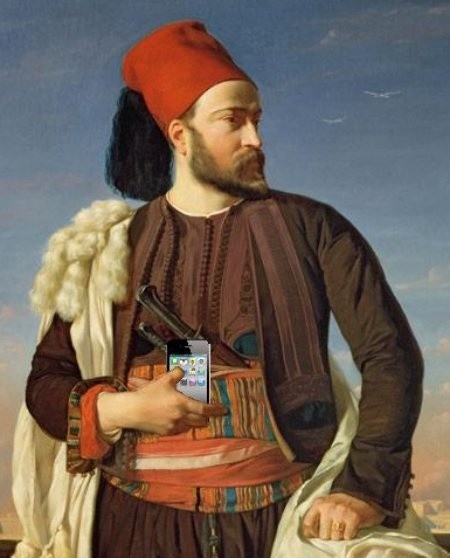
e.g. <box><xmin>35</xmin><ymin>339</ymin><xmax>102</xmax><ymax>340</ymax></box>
<box><xmin>166</xmin><ymin>338</ymin><xmax>211</xmax><ymax>405</ymax></box>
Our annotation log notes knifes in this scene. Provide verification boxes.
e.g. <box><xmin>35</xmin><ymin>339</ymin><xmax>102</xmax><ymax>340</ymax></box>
<box><xmin>134</xmin><ymin>282</ymin><xmax>251</xmax><ymax>366</ymax></box>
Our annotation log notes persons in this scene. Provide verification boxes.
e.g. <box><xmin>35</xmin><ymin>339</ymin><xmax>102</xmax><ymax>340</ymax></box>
<box><xmin>25</xmin><ymin>20</ymin><xmax>425</xmax><ymax>558</ymax></box>
